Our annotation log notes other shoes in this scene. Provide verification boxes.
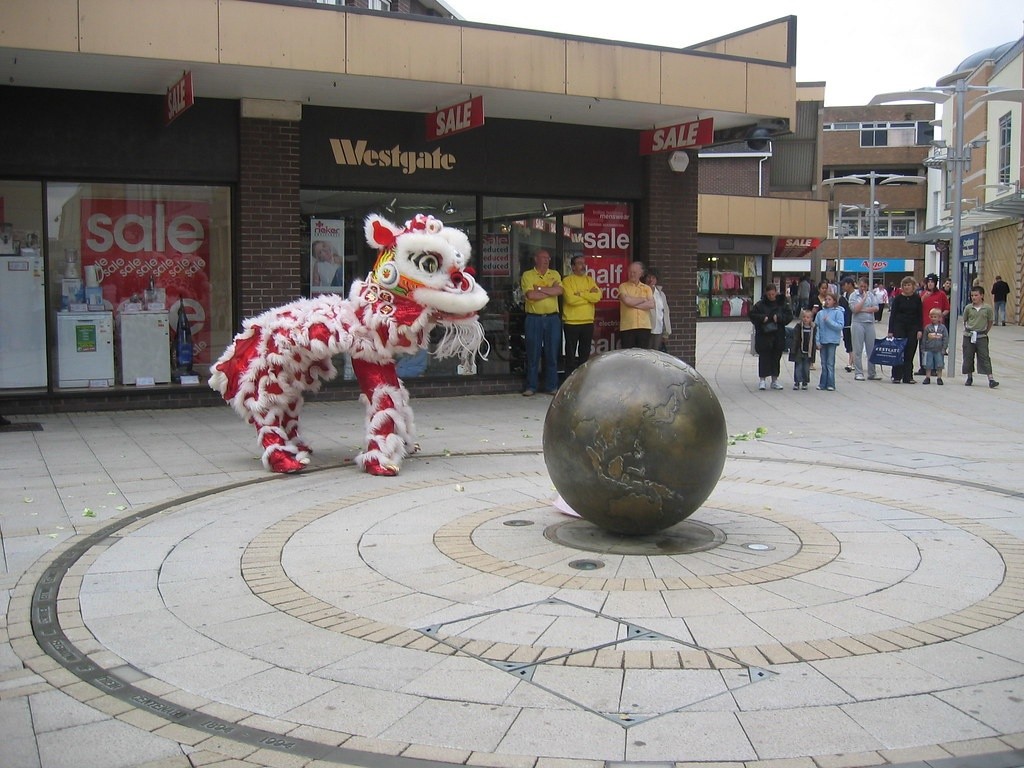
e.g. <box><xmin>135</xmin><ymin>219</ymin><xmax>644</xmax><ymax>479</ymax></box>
<box><xmin>792</xmin><ymin>382</ymin><xmax>800</xmax><ymax>390</ymax></box>
<box><xmin>851</xmin><ymin>363</ymin><xmax>855</xmax><ymax>370</ymax></box>
<box><xmin>816</xmin><ymin>385</ymin><xmax>822</xmax><ymax>390</ymax></box>
<box><xmin>844</xmin><ymin>364</ymin><xmax>851</xmax><ymax>373</ymax></box>
<box><xmin>758</xmin><ymin>381</ymin><xmax>766</xmax><ymax>389</ymax></box>
<box><xmin>964</xmin><ymin>377</ymin><xmax>974</xmax><ymax>386</ymax></box>
<box><xmin>993</xmin><ymin>323</ymin><xmax>998</xmax><ymax>326</ymax></box>
<box><xmin>826</xmin><ymin>386</ymin><xmax>835</xmax><ymax>391</ymax></box>
<box><xmin>874</xmin><ymin>320</ymin><xmax>878</xmax><ymax>323</ymax></box>
<box><xmin>988</xmin><ymin>380</ymin><xmax>999</xmax><ymax>388</ymax></box>
<box><xmin>891</xmin><ymin>379</ymin><xmax>900</xmax><ymax>384</ymax></box>
<box><xmin>770</xmin><ymin>381</ymin><xmax>783</xmax><ymax>390</ymax></box>
<box><xmin>1001</xmin><ymin>321</ymin><xmax>1006</xmax><ymax>326</ymax></box>
<box><xmin>922</xmin><ymin>377</ymin><xmax>930</xmax><ymax>384</ymax></box>
<box><xmin>868</xmin><ymin>374</ymin><xmax>883</xmax><ymax>379</ymax></box>
<box><xmin>801</xmin><ymin>382</ymin><xmax>808</xmax><ymax>389</ymax></box>
<box><xmin>878</xmin><ymin>321</ymin><xmax>882</xmax><ymax>323</ymax></box>
<box><xmin>522</xmin><ymin>390</ymin><xmax>535</xmax><ymax>396</ymax></box>
<box><xmin>903</xmin><ymin>379</ymin><xmax>915</xmax><ymax>384</ymax></box>
<box><xmin>854</xmin><ymin>374</ymin><xmax>866</xmax><ymax>381</ymax></box>
<box><xmin>937</xmin><ymin>378</ymin><xmax>943</xmax><ymax>385</ymax></box>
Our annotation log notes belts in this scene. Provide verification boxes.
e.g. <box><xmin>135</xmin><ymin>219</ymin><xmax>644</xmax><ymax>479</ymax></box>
<box><xmin>527</xmin><ymin>312</ymin><xmax>558</xmax><ymax>317</ymax></box>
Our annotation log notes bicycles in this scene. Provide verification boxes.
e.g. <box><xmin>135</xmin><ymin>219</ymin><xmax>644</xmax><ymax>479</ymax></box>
<box><xmin>494</xmin><ymin>330</ymin><xmax>512</xmax><ymax>362</ymax></box>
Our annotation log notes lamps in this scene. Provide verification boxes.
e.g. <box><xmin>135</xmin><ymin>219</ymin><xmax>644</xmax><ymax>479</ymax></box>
<box><xmin>385</xmin><ymin>197</ymin><xmax>399</xmax><ymax>215</ymax></box>
<box><xmin>542</xmin><ymin>203</ymin><xmax>553</xmax><ymax>217</ymax></box>
<box><xmin>442</xmin><ymin>200</ymin><xmax>455</xmax><ymax>215</ymax></box>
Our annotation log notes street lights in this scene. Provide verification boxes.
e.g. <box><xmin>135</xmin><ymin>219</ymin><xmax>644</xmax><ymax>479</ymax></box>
<box><xmin>863</xmin><ymin>77</ymin><xmax>1024</xmax><ymax>381</ymax></box>
<box><xmin>836</xmin><ymin>202</ymin><xmax>859</xmax><ymax>306</ymax></box>
<box><xmin>820</xmin><ymin>171</ymin><xmax>927</xmax><ymax>291</ymax></box>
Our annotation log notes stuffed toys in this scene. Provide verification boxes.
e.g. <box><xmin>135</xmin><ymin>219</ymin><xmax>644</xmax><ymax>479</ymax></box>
<box><xmin>207</xmin><ymin>214</ymin><xmax>490</xmax><ymax>476</ymax></box>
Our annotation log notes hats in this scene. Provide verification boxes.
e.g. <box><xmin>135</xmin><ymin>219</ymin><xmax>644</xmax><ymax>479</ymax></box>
<box><xmin>924</xmin><ymin>273</ymin><xmax>939</xmax><ymax>281</ymax></box>
<box><xmin>837</xmin><ymin>278</ymin><xmax>854</xmax><ymax>285</ymax></box>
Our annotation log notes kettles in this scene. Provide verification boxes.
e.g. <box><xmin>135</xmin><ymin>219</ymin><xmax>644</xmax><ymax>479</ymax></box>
<box><xmin>85</xmin><ymin>266</ymin><xmax>103</xmax><ymax>287</ymax></box>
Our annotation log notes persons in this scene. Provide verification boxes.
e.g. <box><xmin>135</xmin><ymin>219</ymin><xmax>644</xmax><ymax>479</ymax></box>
<box><xmin>962</xmin><ymin>286</ymin><xmax>999</xmax><ymax>388</ymax></box>
<box><xmin>521</xmin><ymin>250</ymin><xmax>565</xmax><ymax>396</ymax></box>
<box><xmin>873</xmin><ymin>282</ymin><xmax>902</xmax><ymax>323</ymax></box>
<box><xmin>562</xmin><ymin>255</ymin><xmax>602</xmax><ymax>380</ymax></box>
<box><xmin>312</xmin><ymin>241</ymin><xmax>343</xmax><ymax>287</ymax></box>
<box><xmin>888</xmin><ymin>273</ymin><xmax>951</xmax><ymax>385</ymax></box>
<box><xmin>788</xmin><ymin>280</ymin><xmax>845</xmax><ymax>391</ymax></box>
<box><xmin>618</xmin><ymin>261</ymin><xmax>656</xmax><ymax>349</ymax></box>
<box><xmin>838</xmin><ymin>276</ymin><xmax>883</xmax><ymax>381</ymax></box>
<box><xmin>749</xmin><ymin>283</ymin><xmax>794</xmax><ymax>390</ymax></box>
<box><xmin>991</xmin><ymin>276</ymin><xmax>1010</xmax><ymax>326</ymax></box>
<box><xmin>642</xmin><ymin>268</ymin><xmax>671</xmax><ymax>351</ymax></box>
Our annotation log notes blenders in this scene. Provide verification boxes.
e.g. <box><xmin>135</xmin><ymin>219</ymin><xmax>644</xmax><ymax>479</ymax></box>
<box><xmin>63</xmin><ymin>248</ymin><xmax>80</xmax><ymax>278</ymax></box>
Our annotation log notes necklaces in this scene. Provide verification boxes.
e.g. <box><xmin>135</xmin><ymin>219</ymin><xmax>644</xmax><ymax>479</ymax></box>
<box><xmin>534</xmin><ymin>267</ymin><xmax>549</xmax><ymax>279</ymax></box>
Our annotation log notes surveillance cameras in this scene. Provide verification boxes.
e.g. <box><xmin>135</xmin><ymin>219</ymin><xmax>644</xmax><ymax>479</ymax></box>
<box><xmin>744</xmin><ymin>129</ymin><xmax>773</xmax><ymax>151</ymax></box>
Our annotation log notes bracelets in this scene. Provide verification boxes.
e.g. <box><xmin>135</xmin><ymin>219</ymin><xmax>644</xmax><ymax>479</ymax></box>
<box><xmin>538</xmin><ymin>286</ymin><xmax>541</xmax><ymax>290</ymax></box>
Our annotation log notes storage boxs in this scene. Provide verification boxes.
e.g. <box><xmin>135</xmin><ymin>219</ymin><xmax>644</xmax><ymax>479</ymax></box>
<box><xmin>61</xmin><ymin>279</ymin><xmax>84</xmax><ymax>308</ymax></box>
<box><xmin>21</xmin><ymin>247</ymin><xmax>40</xmax><ymax>256</ymax></box>
<box><xmin>85</xmin><ymin>287</ymin><xmax>102</xmax><ymax>305</ymax></box>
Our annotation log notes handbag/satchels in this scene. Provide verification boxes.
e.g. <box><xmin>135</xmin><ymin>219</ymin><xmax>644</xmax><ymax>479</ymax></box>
<box><xmin>868</xmin><ymin>336</ymin><xmax>908</xmax><ymax>366</ymax></box>
